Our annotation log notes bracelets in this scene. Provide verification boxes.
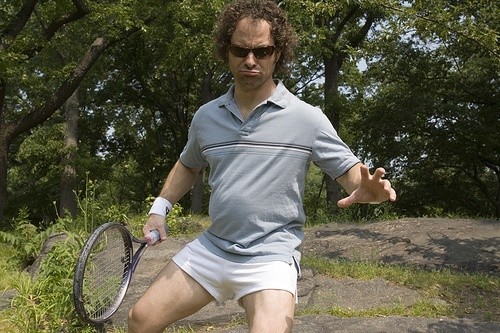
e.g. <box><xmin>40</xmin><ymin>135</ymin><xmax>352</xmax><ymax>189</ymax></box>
<box><xmin>149</xmin><ymin>196</ymin><xmax>173</xmax><ymax>217</ymax></box>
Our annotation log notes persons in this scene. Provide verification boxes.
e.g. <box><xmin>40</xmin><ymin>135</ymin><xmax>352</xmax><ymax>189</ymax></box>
<box><xmin>128</xmin><ymin>0</ymin><xmax>398</xmax><ymax>333</ymax></box>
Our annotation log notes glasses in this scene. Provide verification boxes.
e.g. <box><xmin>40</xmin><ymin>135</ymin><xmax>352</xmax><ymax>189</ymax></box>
<box><xmin>227</xmin><ymin>41</ymin><xmax>278</xmax><ymax>60</ymax></box>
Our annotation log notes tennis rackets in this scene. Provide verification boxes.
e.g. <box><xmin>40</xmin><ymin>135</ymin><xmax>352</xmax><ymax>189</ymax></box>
<box><xmin>73</xmin><ymin>223</ymin><xmax>169</xmax><ymax>326</ymax></box>
